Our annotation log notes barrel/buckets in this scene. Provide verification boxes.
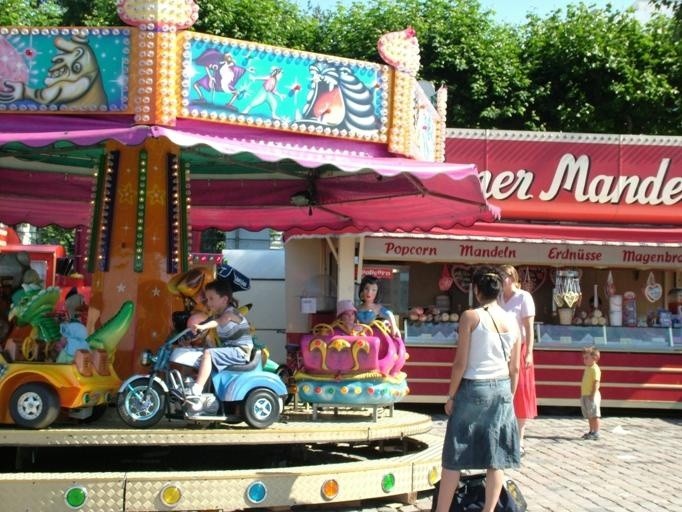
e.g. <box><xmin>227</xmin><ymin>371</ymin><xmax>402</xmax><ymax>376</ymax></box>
<box><xmin>559</xmin><ymin>308</ymin><xmax>573</xmax><ymax>324</ymax></box>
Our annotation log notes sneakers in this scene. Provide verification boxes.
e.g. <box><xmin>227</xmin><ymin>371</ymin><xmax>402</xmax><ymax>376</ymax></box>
<box><xmin>581</xmin><ymin>431</ymin><xmax>600</xmax><ymax>440</ymax></box>
<box><xmin>170</xmin><ymin>385</ymin><xmax>202</xmax><ymax>405</ymax></box>
<box><xmin>519</xmin><ymin>445</ymin><xmax>525</xmax><ymax>456</ymax></box>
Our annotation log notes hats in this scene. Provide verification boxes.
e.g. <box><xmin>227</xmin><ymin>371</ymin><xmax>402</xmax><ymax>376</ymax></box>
<box><xmin>334</xmin><ymin>299</ymin><xmax>358</xmax><ymax>317</ymax></box>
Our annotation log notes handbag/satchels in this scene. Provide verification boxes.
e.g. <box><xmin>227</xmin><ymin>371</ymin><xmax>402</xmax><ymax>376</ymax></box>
<box><xmin>429</xmin><ymin>478</ymin><xmax>514</xmax><ymax>511</ymax></box>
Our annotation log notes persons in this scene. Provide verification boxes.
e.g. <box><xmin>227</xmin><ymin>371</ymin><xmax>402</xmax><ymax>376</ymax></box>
<box><xmin>494</xmin><ymin>265</ymin><xmax>538</xmax><ymax>457</ymax></box>
<box><xmin>585</xmin><ymin>294</ymin><xmax>610</xmax><ymax>326</ymax></box>
<box><xmin>168</xmin><ymin>279</ymin><xmax>255</xmax><ymax>398</ymax></box>
<box><xmin>332</xmin><ymin>297</ymin><xmax>366</xmax><ymax>334</ymax></box>
<box><xmin>433</xmin><ymin>264</ymin><xmax>521</xmax><ymax>511</ymax></box>
<box><xmin>354</xmin><ymin>275</ymin><xmax>402</xmax><ymax>338</ymax></box>
<box><xmin>580</xmin><ymin>346</ymin><xmax>602</xmax><ymax>441</ymax></box>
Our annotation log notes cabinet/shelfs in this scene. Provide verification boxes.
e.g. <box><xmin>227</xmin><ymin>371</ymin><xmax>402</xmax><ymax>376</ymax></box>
<box><xmin>402</xmin><ymin>316</ymin><xmax>682</xmax><ymax>410</ymax></box>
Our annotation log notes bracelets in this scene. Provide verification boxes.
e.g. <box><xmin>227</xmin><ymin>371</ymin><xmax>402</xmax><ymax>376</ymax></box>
<box><xmin>446</xmin><ymin>394</ymin><xmax>455</xmax><ymax>401</ymax></box>
